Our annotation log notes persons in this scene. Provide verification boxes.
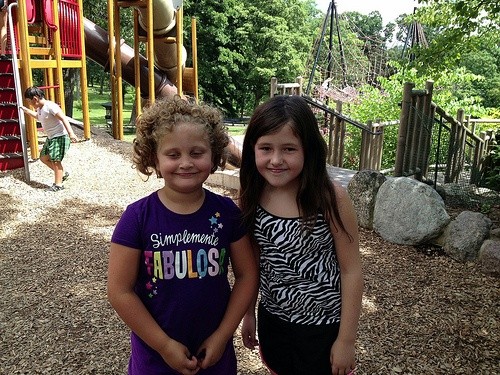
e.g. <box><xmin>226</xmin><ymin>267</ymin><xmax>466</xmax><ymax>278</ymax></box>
<box><xmin>107</xmin><ymin>94</ymin><xmax>258</xmax><ymax>375</ymax></box>
<box><xmin>19</xmin><ymin>86</ymin><xmax>79</xmax><ymax>192</ymax></box>
<box><xmin>240</xmin><ymin>95</ymin><xmax>364</xmax><ymax>375</ymax></box>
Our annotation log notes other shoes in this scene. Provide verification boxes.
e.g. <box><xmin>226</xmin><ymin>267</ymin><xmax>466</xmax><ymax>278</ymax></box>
<box><xmin>62</xmin><ymin>171</ymin><xmax>70</xmax><ymax>184</ymax></box>
<box><xmin>44</xmin><ymin>183</ymin><xmax>64</xmax><ymax>194</ymax></box>
